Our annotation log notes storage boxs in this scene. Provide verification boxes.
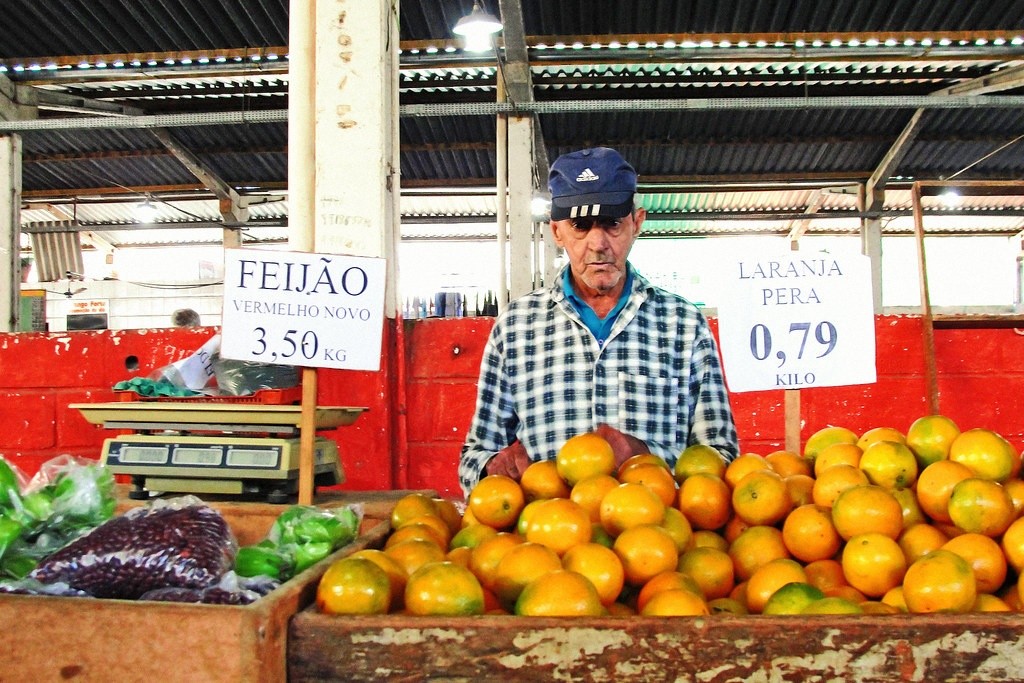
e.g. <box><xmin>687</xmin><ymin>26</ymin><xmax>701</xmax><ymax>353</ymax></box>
<box><xmin>0</xmin><ymin>500</ymin><xmax>397</xmax><ymax>683</ymax></box>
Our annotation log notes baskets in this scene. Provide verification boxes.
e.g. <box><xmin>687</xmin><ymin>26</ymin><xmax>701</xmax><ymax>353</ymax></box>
<box><xmin>112</xmin><ymin>383</ymin><xmax>303</xmax><ymax>404</ymax></box>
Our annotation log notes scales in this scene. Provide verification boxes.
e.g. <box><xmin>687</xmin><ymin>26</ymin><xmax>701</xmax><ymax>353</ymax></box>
<box><xmin>68</xmin><ymin>402</ymin><xmax>370</xmax><ymax>503</ymax></box>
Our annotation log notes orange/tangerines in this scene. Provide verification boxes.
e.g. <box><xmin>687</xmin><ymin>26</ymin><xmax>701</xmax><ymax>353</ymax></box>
<box><xmin>317</xmin><ymin>415</ymin><xmax>1024</xmax><ymax>618</ymax></box>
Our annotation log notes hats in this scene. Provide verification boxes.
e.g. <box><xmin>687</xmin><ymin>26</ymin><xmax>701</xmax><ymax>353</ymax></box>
<box><xmin>547</xmin><ymin>147</ymin><xmax>637</xmax><ymax>222</ymax></box>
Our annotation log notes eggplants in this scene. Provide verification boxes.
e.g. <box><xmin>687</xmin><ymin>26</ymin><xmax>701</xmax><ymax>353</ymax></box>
<box><xmin>0</xmin><ymin>455</ymin><xmax>116</xmax><ymax>589</ymax></box>
<box><xmin>238</xmin><ymin>506</ymin><xmax>358</xmax><ymax>582</ymax></box>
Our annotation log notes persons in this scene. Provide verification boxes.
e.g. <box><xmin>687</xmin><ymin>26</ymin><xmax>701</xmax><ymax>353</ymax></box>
<box><xmin>459</xmin><ymin>146</ymin><xmax>740</xmax><ymax>498</ymax></box>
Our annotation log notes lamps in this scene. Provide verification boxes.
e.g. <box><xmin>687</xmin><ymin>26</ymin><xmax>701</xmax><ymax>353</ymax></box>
<box><xmin>134</xmin><ymin>198</ymin><xmax>158</xmax><ymax>224</ymax></box>
<box><xmin>531</xmin><ymin>188</ymin><xmax>552</xmax><ymax>218</ymax></box>
<box><xmin>452</xmin><ymin>0</ymin><xmax>502</xmax><ymax>52</ymax></box>
